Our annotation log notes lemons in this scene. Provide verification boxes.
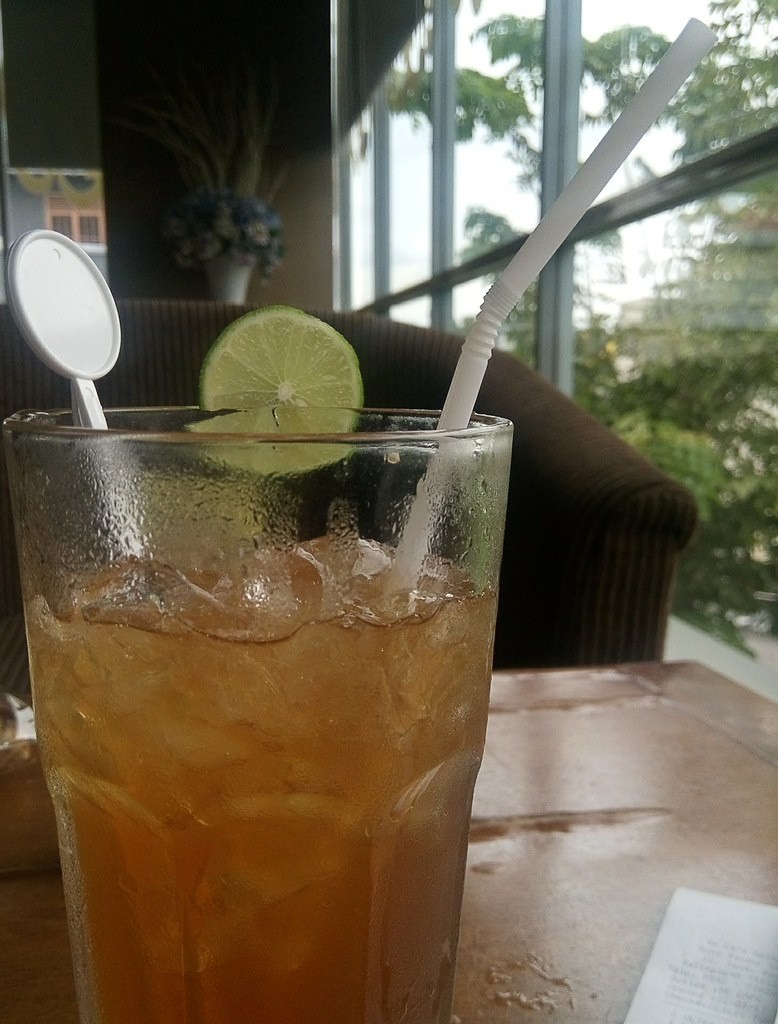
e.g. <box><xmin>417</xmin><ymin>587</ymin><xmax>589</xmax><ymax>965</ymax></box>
<box><xmin>198</xmin><ymin>306</ymin><xmax>365</xmax><ymax>474</ymax></box>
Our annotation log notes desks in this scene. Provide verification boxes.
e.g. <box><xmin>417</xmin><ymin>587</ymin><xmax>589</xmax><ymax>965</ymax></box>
<box><xmin>2</xmin><ymin>665</ymin><xmax>778</xmax><ymax>1022</ymax></box>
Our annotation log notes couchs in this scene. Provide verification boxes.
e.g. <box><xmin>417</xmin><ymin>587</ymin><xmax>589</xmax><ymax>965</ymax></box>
<box><xmin>0</xmin><ymin>298</ymin><xmax>697</xmax><ymax>684</ymax></box>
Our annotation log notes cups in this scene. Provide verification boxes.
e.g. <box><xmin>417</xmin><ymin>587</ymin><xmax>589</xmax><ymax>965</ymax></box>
<box><xmin>5</xmin><ymin>405</ymin><xmax>513</xmax><ymax>1024</ymax></box>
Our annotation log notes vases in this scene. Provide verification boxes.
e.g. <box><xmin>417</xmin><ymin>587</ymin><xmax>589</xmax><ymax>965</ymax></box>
<box><xmin>205</xmin><ymin>263</ymin><xmax>251</xmax><ymax>305</ymax></box>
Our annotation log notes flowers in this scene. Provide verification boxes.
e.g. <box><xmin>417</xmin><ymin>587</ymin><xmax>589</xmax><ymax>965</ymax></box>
<box><xmin>168</xmin><ymin>194</ymin><xmax>287</xmax><ymax>280</ymax></box>
